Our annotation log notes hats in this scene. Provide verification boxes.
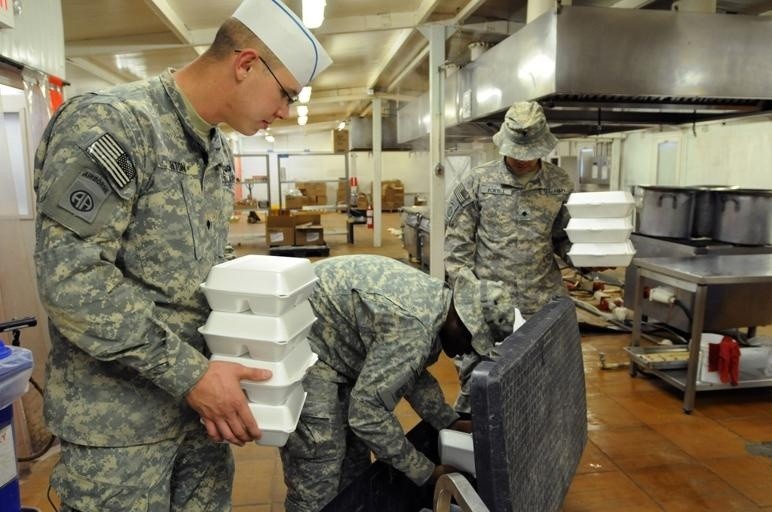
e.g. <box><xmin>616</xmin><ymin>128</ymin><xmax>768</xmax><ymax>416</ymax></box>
<box><xmin>492</xmin><ymin>101</ymin><xmax>558</xmax><ymax>161</ymax></box>
<box><xmin>233</xmin><ymin>0</ymin><xmax>333</xmax><ymax>90</ymax></box>
<box><xmin>454</xmin><ymin>267</ymin><xmax>515</xmax><ymax>359</ymax></box>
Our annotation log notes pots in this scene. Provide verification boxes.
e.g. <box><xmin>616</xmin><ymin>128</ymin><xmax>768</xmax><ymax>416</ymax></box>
<box><xmin>638</xmin><ymin>184</ymin><xmax>772</xmax><ymax>246</ymax></box>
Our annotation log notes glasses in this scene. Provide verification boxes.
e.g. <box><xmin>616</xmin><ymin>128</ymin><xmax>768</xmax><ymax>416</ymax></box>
<box><xmin>235</xmin><ymin>48</ymin><xmax>299</xmax><ymax>105</ymax></box>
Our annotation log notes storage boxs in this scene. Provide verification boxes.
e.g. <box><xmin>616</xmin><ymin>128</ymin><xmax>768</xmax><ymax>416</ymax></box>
<box><xmin>265</xmin><ymin>182</ymin><xmax>328</xmax><ymax>246</ymax></box>
<box><xmin>314</xmin><ymin>293</ymin><xmax>589</xmax><ymax>512</ymax></box>
<box><xmin>335</xmin><ymin>177</ymin><xmax>406</xmax><ymax>213</ymax></box>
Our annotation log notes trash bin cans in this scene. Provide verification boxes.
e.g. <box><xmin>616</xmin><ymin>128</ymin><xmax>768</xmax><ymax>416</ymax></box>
<box><xmin>0</xmin><ymin>340</ymin><xmax>41</xmax><ymax>512</ymax></box>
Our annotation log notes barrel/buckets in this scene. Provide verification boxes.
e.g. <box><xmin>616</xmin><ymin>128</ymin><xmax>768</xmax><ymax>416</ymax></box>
<box><xmin>699</xmin><ymin>332</ymin><xmax>736</xmax><ymax>388</ymax></box>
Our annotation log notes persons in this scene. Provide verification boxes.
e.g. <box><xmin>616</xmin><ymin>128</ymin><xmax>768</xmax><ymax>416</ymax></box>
<box><xmin>443</xmin><ymin>102</ymin><xmax>579</xmax><ymax>424</ymax></box>
<box><xmin>31</xmin><ymin>0</ymin><xmax>334</xmax><ymax>512</ymax></box>
<box><xmin>278</xmin><ymin>250</ymin><xmax>517</xmax><ymax>512</ymax></box>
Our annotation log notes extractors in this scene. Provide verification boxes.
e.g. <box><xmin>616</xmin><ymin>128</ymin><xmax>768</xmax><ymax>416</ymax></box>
<box><xmin>396</xmin><ymin>5</ymin><xmax>772</xmax><ymax>151</ymax></box>
<box><xmin>348</xmin><ymin>113</ymin><xmax>413</xmax><ymax>153</ymax></box>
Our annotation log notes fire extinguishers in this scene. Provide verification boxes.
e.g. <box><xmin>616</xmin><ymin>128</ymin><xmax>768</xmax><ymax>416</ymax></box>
<box><xmin>367</xmin><ymin>203</ymin><xmax>373</xmax><ymax>229</ymax></box>
